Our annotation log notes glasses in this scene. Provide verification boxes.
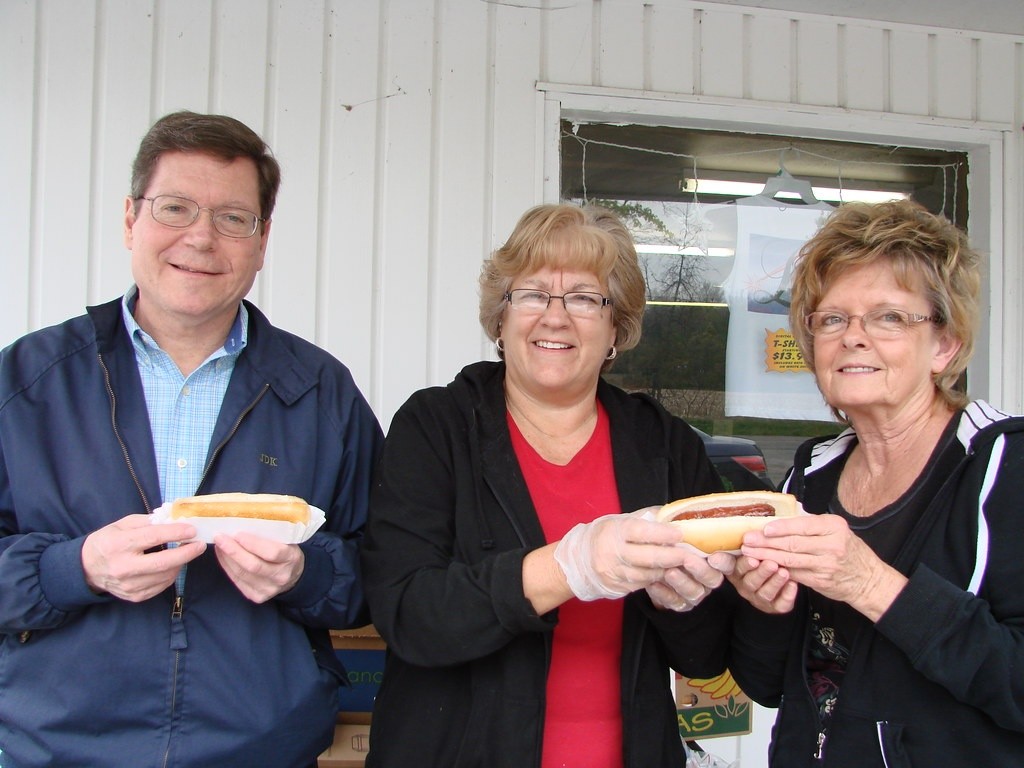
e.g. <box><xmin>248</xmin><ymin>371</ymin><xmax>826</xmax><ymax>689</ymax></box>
<box><xmin>133</xmin><ymin>196</ymin><xmax>265</xmax><ymax>238</ymax></box>
<box><xmin>504</xmin><ymin>289</ymin><xmax>613</xmax><ymax>320</ymax></box>
<box><xmin>803</xmin><ymin>310</ymin><xmax>941</xmax><ymax>339</ymax></box>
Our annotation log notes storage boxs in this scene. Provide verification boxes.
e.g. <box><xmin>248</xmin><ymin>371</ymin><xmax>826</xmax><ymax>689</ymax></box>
<box><xmin>670</xmin><ymin>669</ymin><xmax>753</xmax><ymax>742</ymax></box>
<box><xmin>315</xmin><ymin>724</ymin><xmax>371</xmax><ymax>768</ymax></box>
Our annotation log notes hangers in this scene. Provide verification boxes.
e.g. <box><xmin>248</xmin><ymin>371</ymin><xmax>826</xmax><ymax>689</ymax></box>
<box><xmin>762</xmin><ymin>146</ymin><xmax>820</xmax><ymax>204</ymax></box>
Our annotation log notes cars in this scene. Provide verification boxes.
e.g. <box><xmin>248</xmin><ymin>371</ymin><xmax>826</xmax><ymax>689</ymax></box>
<box><xmin>690</xmin><ymin>426</ymin><xmax>776</xmax><ymax>492</ymax></box>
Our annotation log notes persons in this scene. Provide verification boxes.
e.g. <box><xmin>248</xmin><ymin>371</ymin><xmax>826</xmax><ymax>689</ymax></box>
<box><xmin>358</xmin><ymin>202</ymin><xmax>736</xmax><ymax>768</ymax></box>
<box><xmin>0</xmin><ymin>111</ymin><xmax>382</xmax><ymax>768</ymax></box>
<box><xmin>728</xmin><ymin>202</ymin><xmax>1024</xmax><ymax>767</ymax></box>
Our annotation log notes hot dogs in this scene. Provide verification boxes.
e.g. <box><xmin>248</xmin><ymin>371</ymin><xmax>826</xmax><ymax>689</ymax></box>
<box><xmin>655</xmin><ymin>490</ymin><xmax>798</xmax><ymax>554</ymax></box>
<box><xmin>171</xmin><ymin>492</ymin><xmax>311</xmax><ymax>526</ymax></box>
